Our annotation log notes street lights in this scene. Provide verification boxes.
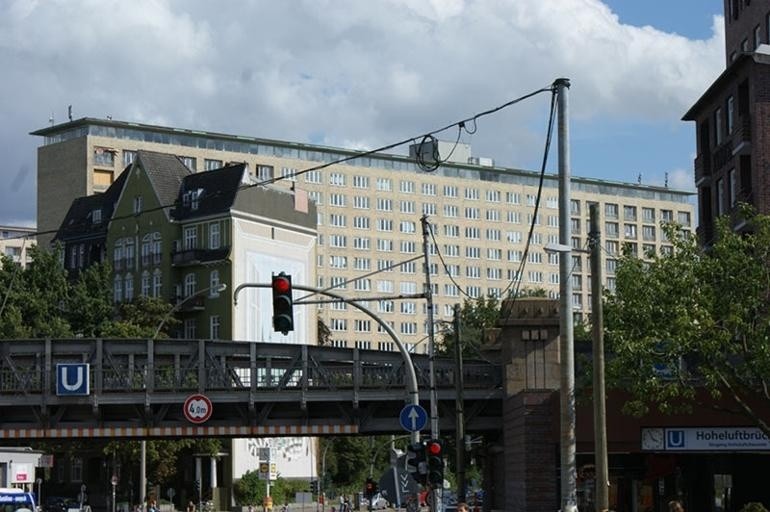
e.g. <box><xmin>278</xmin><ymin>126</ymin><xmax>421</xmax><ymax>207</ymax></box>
<box><xmin>153</xmin><ymin>282</ymin><xmax>227</xmax><ymax>341</ymax></box>
<box><xmin>545</xmin><ymin>202</ymin><xmax>610</xmax><ymax>512</ymax></box>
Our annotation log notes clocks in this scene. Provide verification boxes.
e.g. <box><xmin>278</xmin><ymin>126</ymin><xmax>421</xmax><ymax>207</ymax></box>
<box><xmin>639</xmin><ymin>425</ymin><xmax>666</xmax><ymax>451</ymax></box>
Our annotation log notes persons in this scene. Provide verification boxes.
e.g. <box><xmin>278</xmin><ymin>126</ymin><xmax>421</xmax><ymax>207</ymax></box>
<box><xmin>146</xmin><ymin>501</ymin><xmax>156</xmax><ymax>511</ymax></box>
<box><xmin>186</xmin><ymin>500</ymin><xmax>196</xmax><ymax>512</ymax></box>
<box><xmin>152</xmin><ymin>498</ymin><xmax>160</xmax><ymax>511</ymax></box>
<box><xmin>456</xmin><ymin>502</ymin><xmax>471</xmax><ymax>511</ymax></box>
<box><xmin>339</xmin><ymin>492</ymin><xmax>352</xmax><ymax>511</ymax></box>
<box><xmin>668</xmin><ymin>501</ymin><xmax>684</xmax><ymax>511</ymax></box>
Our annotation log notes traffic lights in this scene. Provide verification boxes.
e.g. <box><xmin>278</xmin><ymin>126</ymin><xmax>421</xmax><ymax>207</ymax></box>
<box><xmin>272</xmin><ymin>275</ymin><xmax>294</xmax><ymax>333</ymax></box>
<box><xmin>427</xmin><ymin>439</ymin><xmax>443</xmax><ymax>485</ymax></box>
<box><xmin>363</xmin><ymin>478</ymin><xmax>378</xmax><ymax>500</ymax></box>
<box><xmin>407</xmin><ymin>441</ymin><xmax>428</xmax><ymax>483</ymax></box>
